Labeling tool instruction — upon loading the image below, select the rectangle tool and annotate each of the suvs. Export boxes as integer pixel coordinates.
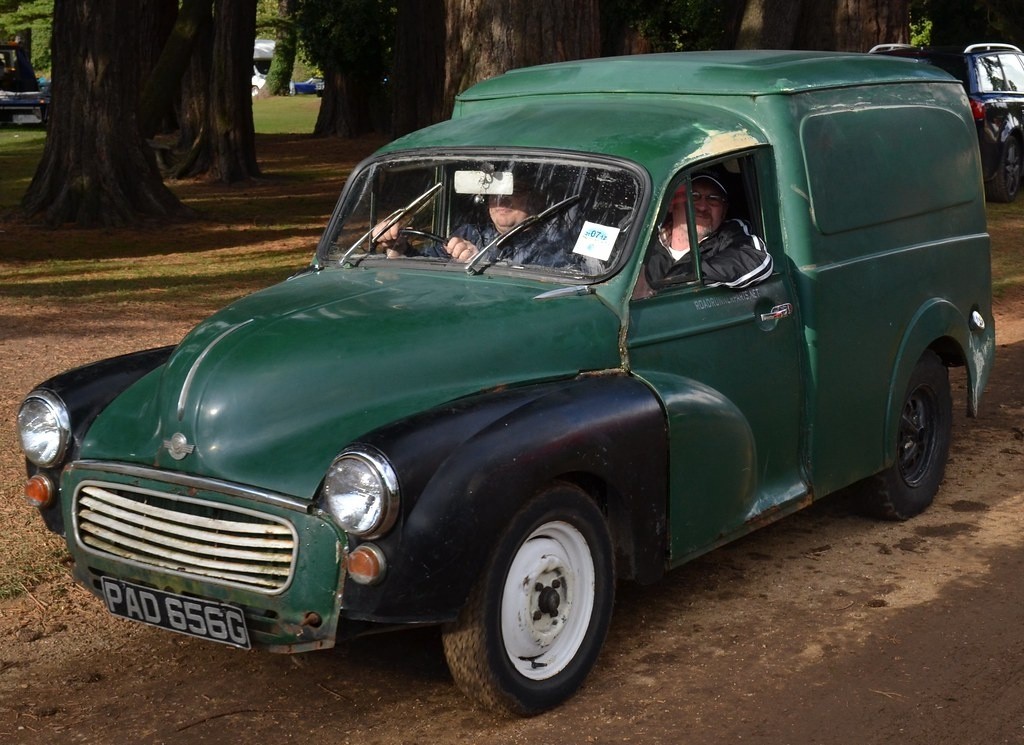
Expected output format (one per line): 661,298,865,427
868,43,1024,203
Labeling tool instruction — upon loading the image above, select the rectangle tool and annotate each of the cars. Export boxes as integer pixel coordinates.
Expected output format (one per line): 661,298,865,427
294,77,324,95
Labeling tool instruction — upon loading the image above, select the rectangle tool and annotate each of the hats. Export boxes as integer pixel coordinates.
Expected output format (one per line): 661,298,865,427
675,167,728,194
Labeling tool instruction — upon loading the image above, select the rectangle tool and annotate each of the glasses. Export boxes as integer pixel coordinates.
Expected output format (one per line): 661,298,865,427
690,190,726,207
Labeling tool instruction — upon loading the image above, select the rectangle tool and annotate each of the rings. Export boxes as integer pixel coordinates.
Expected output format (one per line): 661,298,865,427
467,248,472,253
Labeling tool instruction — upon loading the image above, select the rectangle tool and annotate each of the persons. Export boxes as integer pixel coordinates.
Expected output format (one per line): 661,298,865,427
372,170,589,276
638,168,774,289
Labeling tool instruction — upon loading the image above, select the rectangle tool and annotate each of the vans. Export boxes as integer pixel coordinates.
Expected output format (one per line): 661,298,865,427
17,49,996,719
251,40,295,97
0,42,50,115
